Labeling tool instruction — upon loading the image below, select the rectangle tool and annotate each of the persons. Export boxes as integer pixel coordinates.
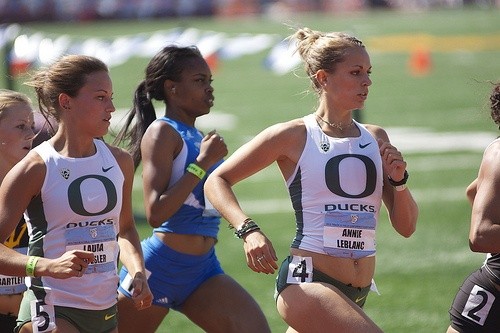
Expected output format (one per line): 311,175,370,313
201,20,420,332
445,77,500,333
1,44,272,333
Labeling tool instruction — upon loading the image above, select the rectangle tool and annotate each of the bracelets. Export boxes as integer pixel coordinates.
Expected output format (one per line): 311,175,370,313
387,170,409,187
391,183,407,191
186,162,206,180
226,218,260,240
25,255,42,278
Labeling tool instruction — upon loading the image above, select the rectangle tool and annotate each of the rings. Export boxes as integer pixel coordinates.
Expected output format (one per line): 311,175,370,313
256,255,265,263
79,265,83,271
78,271,80,277
140,300,144,309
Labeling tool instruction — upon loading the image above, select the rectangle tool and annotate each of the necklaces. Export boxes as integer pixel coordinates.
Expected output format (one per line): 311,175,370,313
314,110,355,130
